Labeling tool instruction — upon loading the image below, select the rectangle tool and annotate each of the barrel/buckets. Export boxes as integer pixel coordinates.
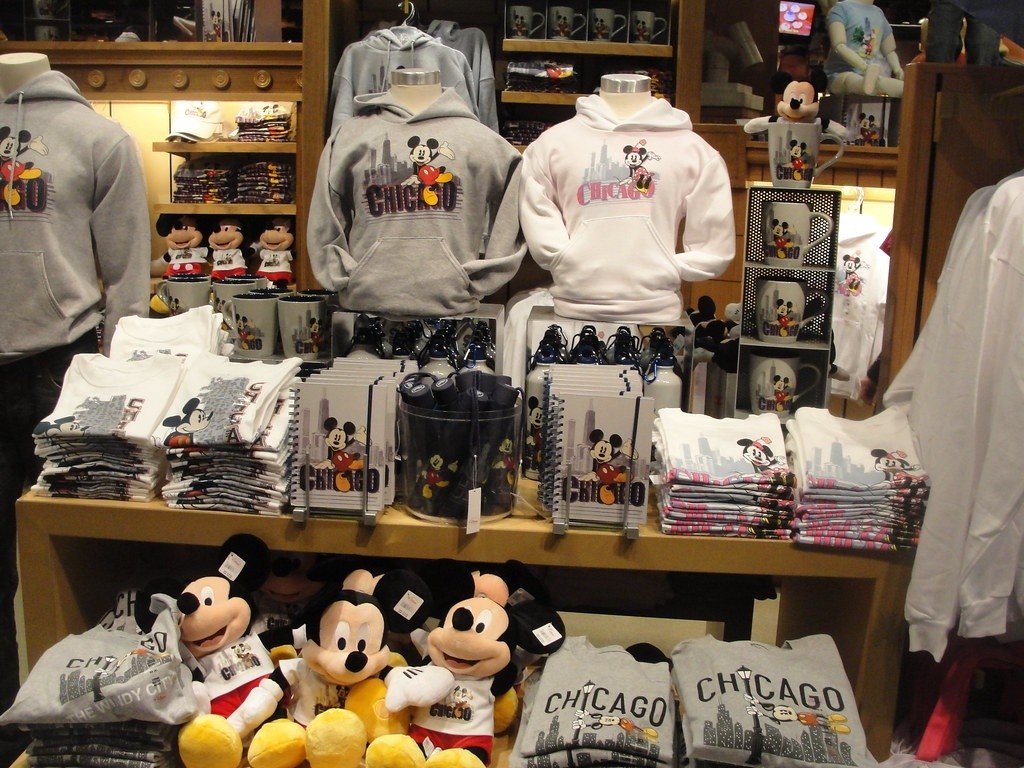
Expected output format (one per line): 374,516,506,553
397,398,520,526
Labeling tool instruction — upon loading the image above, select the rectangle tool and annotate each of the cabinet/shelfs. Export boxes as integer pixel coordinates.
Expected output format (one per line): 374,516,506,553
736,185,839,419
157,48,325,298
501,0,704,155
16,466,914,761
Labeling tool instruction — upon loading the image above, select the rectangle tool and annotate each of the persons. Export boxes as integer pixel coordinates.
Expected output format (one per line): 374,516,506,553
824,0,908,97
517,74,735,323
0,52,152,729
306,68,526,317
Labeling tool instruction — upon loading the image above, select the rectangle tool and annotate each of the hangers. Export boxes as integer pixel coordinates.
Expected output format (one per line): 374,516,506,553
342,4,481,70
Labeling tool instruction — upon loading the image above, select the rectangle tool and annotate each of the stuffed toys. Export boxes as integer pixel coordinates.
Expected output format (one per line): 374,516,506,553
743,69,850,139
150,214,300,291
133,532,565,768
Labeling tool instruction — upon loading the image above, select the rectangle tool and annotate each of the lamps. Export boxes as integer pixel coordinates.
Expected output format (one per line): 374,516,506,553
701,16,769,112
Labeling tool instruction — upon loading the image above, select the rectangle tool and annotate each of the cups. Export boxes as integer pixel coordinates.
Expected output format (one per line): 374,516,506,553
760,200,833,268
548,6,586,42
31,0,69,19
589,7,628,42
507,5,546,39
748,350,823,418
157,270,342,363
33,26,62,42
753,277,829,347
630,10,668,44
768,121,845,193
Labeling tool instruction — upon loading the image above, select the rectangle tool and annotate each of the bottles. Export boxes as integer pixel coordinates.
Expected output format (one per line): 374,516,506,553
347,312,499,393
525,322,682,483
